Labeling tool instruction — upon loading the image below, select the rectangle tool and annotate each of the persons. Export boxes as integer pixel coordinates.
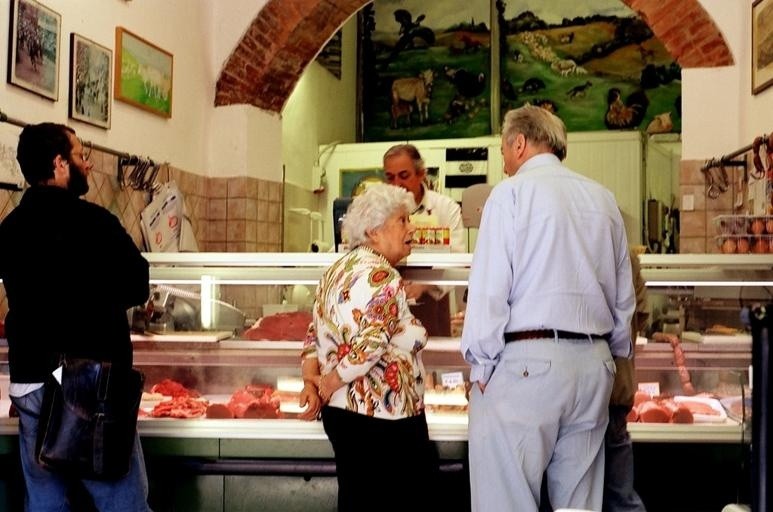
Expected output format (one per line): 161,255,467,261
0,122,151,510
296,179,435,512
382,145,466,253
459,104,638,512
599,239,646,510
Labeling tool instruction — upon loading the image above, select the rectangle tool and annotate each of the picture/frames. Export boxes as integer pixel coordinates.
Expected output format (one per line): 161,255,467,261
8,0,61,104
69,32,114,131
750,0,772,95
114,27,174,120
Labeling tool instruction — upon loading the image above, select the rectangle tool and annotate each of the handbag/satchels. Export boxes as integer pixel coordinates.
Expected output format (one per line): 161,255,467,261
36,353,146,482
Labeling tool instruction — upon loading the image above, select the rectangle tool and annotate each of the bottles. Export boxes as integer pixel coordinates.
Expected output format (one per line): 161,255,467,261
421,228,435,244
411,229,422,244
435,228,449,245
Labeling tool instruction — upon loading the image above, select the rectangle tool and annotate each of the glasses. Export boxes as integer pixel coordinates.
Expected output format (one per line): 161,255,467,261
71,146,93,160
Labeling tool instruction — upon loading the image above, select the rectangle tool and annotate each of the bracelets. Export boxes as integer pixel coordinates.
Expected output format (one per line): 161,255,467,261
316,376,334,402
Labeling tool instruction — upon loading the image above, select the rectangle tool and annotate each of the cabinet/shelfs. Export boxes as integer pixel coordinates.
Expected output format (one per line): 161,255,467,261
0,250,773,512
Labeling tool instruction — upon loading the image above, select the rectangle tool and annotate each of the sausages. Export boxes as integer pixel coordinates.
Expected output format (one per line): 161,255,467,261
651,331,694,396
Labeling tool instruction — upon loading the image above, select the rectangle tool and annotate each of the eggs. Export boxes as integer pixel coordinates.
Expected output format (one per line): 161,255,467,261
750,220,764,235
768,239,772,252
764,219,773,234
722,238,735,253
736,238,748,252
753,239,768,253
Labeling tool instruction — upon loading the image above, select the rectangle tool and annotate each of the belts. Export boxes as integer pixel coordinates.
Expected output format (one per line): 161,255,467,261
504,326,599,342
